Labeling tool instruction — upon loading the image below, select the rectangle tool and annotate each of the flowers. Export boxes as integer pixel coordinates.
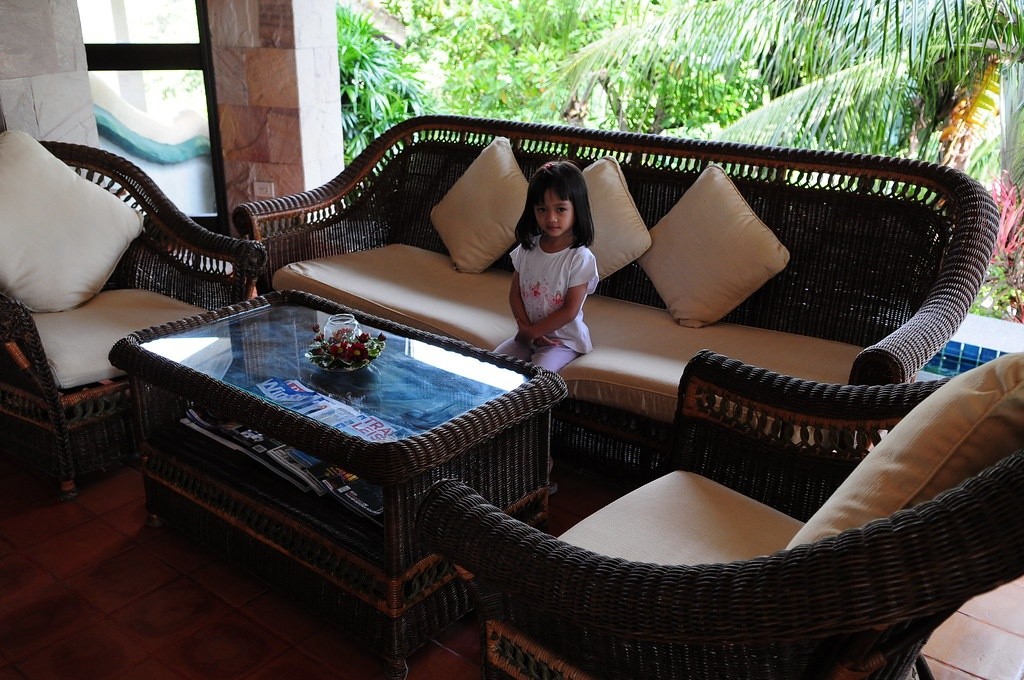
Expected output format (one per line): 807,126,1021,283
303,324,387,372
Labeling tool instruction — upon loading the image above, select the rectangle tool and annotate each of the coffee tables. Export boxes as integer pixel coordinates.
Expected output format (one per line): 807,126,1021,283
107,286,570,680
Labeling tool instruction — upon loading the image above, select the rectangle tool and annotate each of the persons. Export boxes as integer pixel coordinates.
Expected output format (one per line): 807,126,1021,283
492,160,599,386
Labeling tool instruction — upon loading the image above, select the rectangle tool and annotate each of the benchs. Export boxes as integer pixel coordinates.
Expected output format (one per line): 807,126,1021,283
229,116,999,482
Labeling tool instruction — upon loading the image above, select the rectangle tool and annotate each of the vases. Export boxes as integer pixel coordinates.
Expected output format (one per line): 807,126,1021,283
324,314,362,342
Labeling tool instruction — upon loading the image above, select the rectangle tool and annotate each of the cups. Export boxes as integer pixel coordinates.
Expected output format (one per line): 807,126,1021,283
324,314,363,344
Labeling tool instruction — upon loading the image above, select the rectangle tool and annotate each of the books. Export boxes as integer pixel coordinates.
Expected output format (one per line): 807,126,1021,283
180,376,468,530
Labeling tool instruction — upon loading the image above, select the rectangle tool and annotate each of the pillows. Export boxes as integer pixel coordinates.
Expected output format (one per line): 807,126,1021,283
783,352,1024,551
581,155,653,282
430,136,529,275
0,129,144,313
635,164,791,328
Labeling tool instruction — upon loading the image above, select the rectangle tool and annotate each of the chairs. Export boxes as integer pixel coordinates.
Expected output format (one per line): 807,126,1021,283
409,348,1024,680
1,140,269,503
